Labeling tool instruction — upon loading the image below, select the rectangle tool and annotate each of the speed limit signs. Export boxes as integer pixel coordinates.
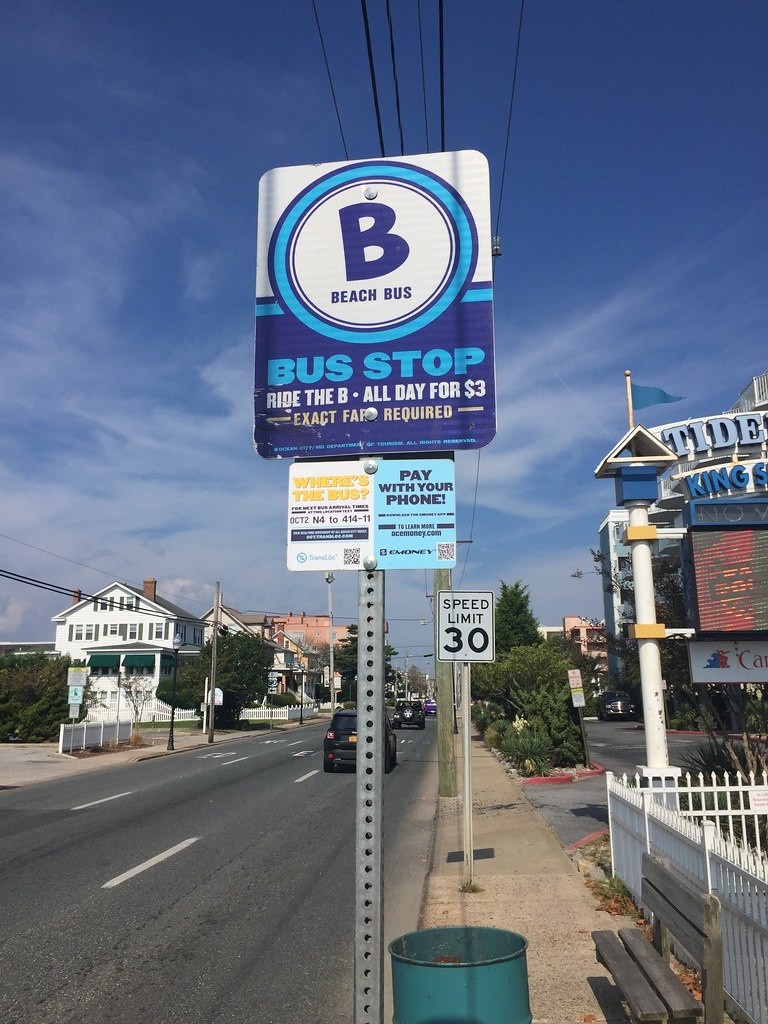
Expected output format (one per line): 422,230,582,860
434,589,496,664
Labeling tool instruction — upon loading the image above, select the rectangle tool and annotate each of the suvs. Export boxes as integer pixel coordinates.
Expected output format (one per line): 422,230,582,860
323,707,399,774
392,700,427,729
597,690,637,721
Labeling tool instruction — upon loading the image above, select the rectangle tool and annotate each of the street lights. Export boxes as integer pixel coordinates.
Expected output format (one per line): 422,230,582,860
165,632,183,750
298,664,306,725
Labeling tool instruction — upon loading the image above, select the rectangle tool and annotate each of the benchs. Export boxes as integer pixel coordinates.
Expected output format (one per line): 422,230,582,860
591,851,723,1023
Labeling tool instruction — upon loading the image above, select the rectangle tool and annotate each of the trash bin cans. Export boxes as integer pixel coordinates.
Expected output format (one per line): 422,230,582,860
388,923,532,1024
